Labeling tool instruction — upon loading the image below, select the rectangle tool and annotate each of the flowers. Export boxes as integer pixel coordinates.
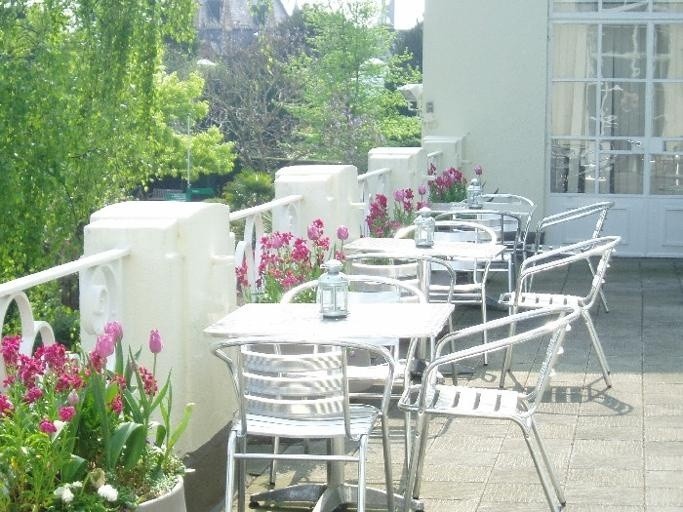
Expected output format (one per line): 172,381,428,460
236,219,352,304
0,323,183,512
427,164,483,202
366,187,431,236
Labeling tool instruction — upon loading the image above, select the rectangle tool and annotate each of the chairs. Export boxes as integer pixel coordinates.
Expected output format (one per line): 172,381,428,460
457,192,530,273
435,210,523,304
213,334,395,508
400,305,581,511
342,251,463,387
278,275,435,452
528,198,615,315
390,220,499,369
498,236,623,389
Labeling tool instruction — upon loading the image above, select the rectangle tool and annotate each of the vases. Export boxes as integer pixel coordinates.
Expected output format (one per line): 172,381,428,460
132,476,185,511
